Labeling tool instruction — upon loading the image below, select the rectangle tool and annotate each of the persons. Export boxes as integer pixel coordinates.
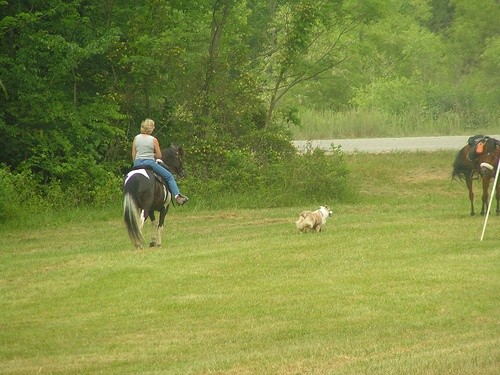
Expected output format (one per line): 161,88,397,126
131,118,189,206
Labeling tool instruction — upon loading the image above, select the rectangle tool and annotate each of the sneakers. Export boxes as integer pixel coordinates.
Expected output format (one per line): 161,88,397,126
175,194,190,206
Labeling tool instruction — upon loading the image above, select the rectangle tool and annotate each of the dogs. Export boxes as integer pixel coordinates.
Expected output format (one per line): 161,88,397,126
296,205,333,234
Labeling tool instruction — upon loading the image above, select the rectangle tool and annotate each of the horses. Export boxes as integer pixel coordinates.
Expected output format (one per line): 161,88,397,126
451,135,500,216
123,143,185,251
